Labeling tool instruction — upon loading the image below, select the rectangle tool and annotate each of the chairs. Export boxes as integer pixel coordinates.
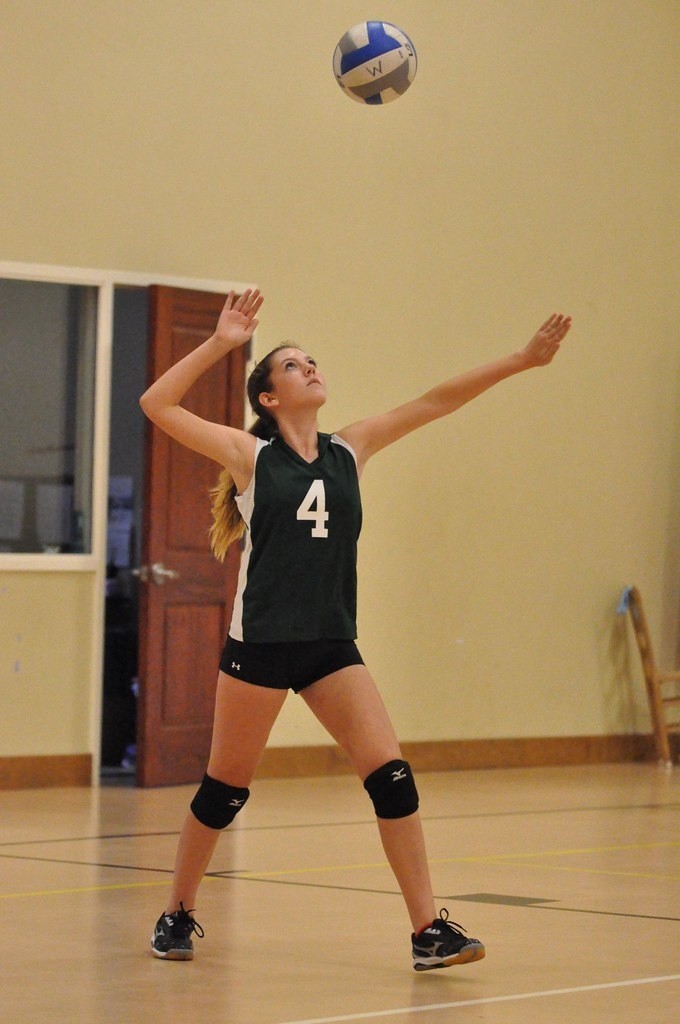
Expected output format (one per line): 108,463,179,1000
627,586,680,769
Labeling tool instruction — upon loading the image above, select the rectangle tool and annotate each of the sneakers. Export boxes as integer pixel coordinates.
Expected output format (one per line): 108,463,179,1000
411,908,485,971
151,901,204,960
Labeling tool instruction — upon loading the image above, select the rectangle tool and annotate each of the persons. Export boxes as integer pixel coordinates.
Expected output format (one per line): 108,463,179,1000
138,287,573,971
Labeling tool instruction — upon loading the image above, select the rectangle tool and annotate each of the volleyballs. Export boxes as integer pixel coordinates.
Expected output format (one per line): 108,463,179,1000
332,20,418,105
